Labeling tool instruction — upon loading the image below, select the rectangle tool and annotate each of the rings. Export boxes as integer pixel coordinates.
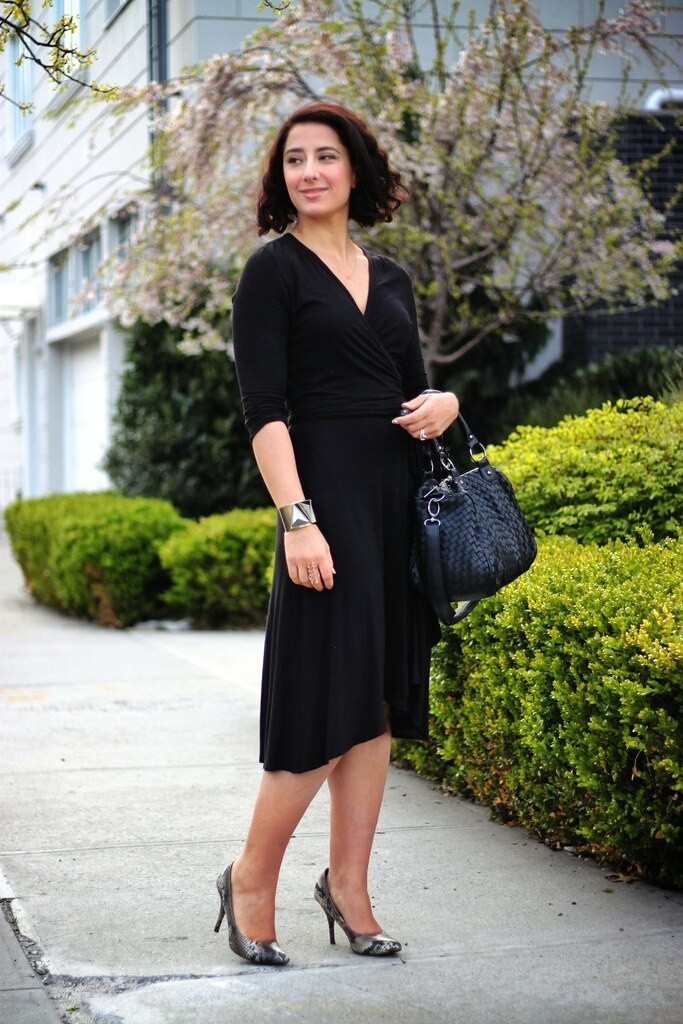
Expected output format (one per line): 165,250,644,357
419,429,426,441
307,560,318,584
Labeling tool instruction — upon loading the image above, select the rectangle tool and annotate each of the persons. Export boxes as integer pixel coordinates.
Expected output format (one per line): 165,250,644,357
216,101,464,970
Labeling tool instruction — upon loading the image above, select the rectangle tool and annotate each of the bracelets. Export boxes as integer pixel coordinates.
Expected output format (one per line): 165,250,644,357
278,499,319,533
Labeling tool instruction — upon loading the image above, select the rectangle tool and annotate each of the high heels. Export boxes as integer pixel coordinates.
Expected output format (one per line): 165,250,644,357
213,861,290,966
314,868,401,957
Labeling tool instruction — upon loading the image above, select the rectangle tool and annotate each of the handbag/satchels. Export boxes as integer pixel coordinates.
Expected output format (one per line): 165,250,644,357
408,387,539,626
